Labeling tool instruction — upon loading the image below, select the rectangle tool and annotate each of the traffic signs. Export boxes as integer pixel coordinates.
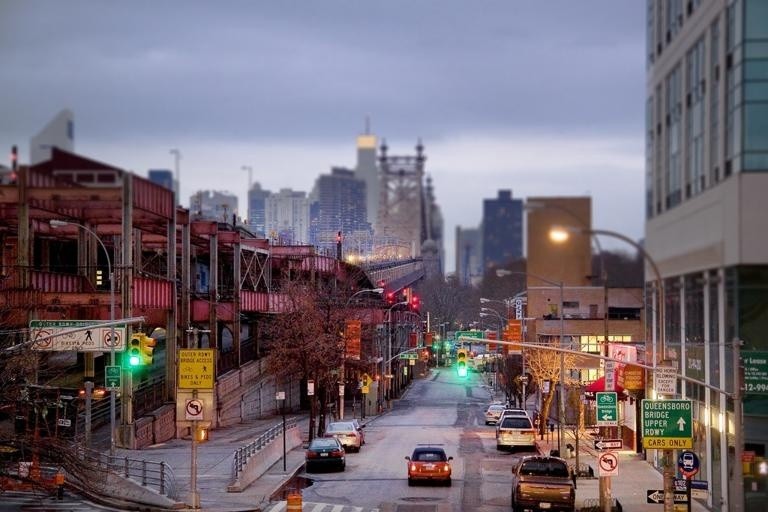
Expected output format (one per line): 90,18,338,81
596,391,618,427
594,439,623,450
105,366,122,392
640,399,694,449
647,479,709,512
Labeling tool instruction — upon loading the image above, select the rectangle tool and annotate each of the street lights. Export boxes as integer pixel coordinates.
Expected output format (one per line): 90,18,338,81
337,288,384,421
547,224,675,512
478,297,527,411
496,268,568,459
50,219,116,463
381,301,408,409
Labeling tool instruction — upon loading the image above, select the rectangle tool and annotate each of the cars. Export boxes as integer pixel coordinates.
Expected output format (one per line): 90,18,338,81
404,446,453,487
323,418,366,454
483,404,537,452
510,454,576,512
303,436,347,475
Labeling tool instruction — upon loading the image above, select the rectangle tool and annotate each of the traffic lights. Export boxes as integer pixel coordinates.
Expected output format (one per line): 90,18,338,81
457,348,468,378
129,334,142,368
